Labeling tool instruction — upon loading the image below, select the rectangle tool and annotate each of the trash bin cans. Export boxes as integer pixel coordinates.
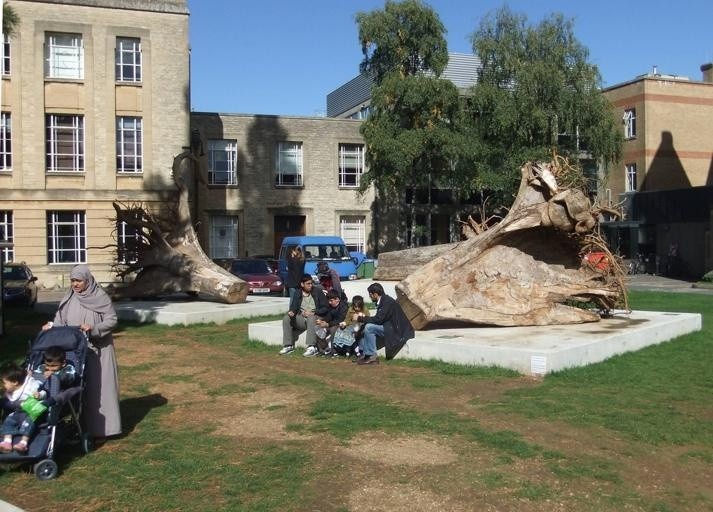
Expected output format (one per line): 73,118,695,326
357,260,374,279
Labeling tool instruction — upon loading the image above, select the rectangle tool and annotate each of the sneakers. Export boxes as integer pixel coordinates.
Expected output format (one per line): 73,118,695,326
277,344,295,355
0,440,27,454
301,346,319,357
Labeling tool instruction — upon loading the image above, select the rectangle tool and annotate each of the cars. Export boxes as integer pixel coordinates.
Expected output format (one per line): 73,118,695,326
1,260,39,309
213,254,285,296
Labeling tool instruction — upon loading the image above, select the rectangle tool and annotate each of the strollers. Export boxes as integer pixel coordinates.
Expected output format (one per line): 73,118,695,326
0,325,95,482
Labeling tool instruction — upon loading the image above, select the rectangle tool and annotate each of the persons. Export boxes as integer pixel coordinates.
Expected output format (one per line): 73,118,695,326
331,295,370,360
27,345,77,389
287,247,306,307
351,282,415,365
315,288,348,357
0,365,50,453
42,264,122,445
277,273,330,358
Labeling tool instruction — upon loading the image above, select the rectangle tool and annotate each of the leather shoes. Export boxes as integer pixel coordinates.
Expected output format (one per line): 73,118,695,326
350,353,378,366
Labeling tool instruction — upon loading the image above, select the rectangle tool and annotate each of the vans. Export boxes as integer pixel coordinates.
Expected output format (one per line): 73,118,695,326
278,234,364,296
